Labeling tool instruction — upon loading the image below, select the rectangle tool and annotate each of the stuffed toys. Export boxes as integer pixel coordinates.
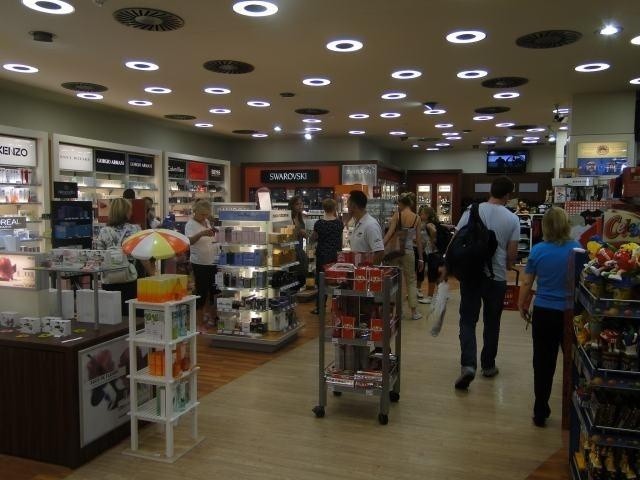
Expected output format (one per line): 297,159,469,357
569,236,640,480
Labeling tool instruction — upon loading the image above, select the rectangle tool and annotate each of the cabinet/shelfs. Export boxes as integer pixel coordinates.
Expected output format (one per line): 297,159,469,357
562,246,640,479
55,136,161,287
367,179,399,238
166,152,228,293
1,248,199,464
248,186,336,210
297,211,324,297
211,205,305,347
0,128,47,254
515,214,544,267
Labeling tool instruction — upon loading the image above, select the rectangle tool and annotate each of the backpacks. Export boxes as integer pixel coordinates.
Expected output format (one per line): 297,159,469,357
101,223,138,285
425,220,453,255
443,202,498,282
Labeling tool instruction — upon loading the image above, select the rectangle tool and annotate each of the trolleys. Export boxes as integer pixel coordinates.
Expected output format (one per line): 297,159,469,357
311,266,402,424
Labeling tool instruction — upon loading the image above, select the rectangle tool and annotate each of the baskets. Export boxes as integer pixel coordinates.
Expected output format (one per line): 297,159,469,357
502,268,535,312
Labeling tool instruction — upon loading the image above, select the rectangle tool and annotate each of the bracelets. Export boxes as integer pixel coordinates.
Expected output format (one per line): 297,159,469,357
419,260,424,262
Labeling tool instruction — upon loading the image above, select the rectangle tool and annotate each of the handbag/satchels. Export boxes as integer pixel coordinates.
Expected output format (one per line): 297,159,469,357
426,279,449,337
384,210,408,262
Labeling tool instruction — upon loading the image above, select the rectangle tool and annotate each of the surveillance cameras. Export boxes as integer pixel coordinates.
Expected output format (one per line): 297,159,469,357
553,113,564,119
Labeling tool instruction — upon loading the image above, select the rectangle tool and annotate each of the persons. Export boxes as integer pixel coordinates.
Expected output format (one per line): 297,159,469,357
505,199,519,214
310,198,344,314
438,175,520,390
346,190,385,265
381,192,425,320
185,200,217,324
286,196,309,292
518,207,585,427
417,207,439,304
418,205,430,298
98,189,161,317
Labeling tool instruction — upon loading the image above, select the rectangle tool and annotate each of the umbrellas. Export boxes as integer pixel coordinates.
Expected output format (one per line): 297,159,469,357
121,228,191,278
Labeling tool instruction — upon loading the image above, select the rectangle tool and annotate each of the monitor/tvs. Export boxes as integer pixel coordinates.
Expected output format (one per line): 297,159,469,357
486,149,527,175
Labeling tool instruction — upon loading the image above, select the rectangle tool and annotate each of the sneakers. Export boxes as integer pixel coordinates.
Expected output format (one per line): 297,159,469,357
412,312,422,320
417,296,433,304
416,288,424,298
455,366,476,390
481,368,498,377
